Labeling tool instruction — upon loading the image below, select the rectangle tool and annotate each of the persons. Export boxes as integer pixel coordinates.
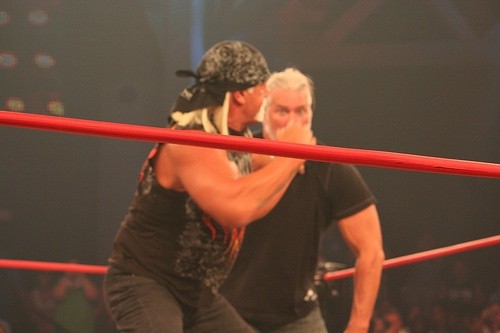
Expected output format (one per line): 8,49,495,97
0,256,113,333
371,259,500,333
103,39,317,333
218,66,385,333
